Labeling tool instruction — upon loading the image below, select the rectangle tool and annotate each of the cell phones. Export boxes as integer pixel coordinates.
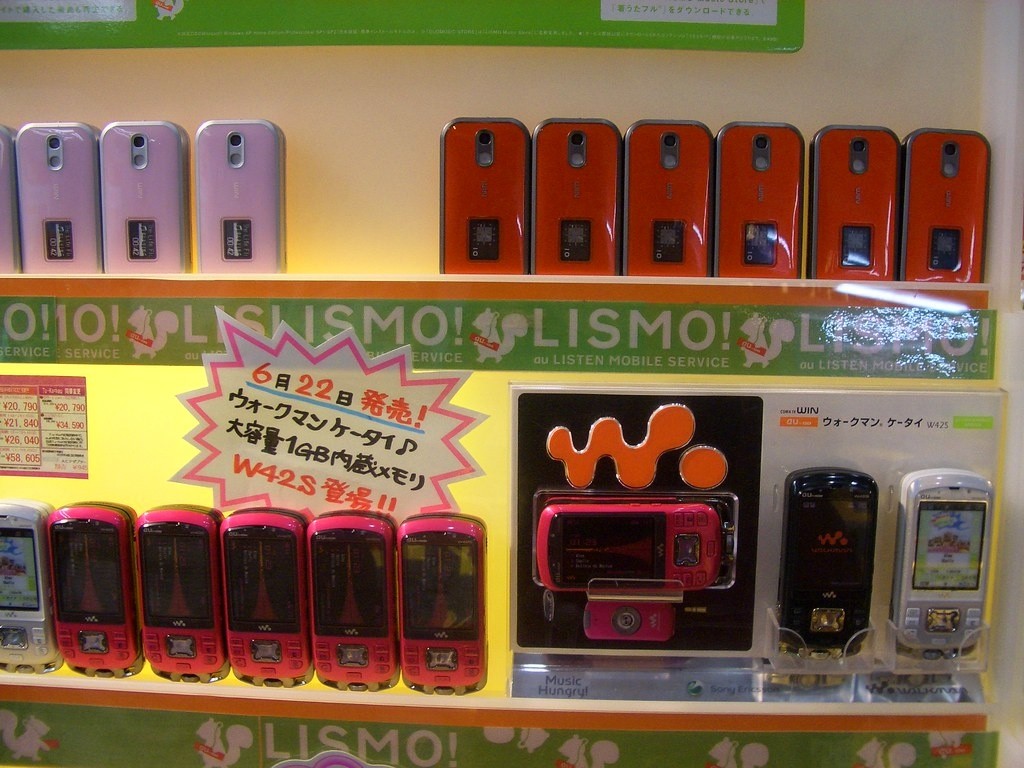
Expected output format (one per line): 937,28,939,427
777,467,880,649
0,499,486,697
1,118,287,277
892,468,997,648
536,496,720,595
435,119,990,285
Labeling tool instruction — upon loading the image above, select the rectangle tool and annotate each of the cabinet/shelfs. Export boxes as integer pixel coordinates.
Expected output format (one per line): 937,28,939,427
0,273,1012,767
